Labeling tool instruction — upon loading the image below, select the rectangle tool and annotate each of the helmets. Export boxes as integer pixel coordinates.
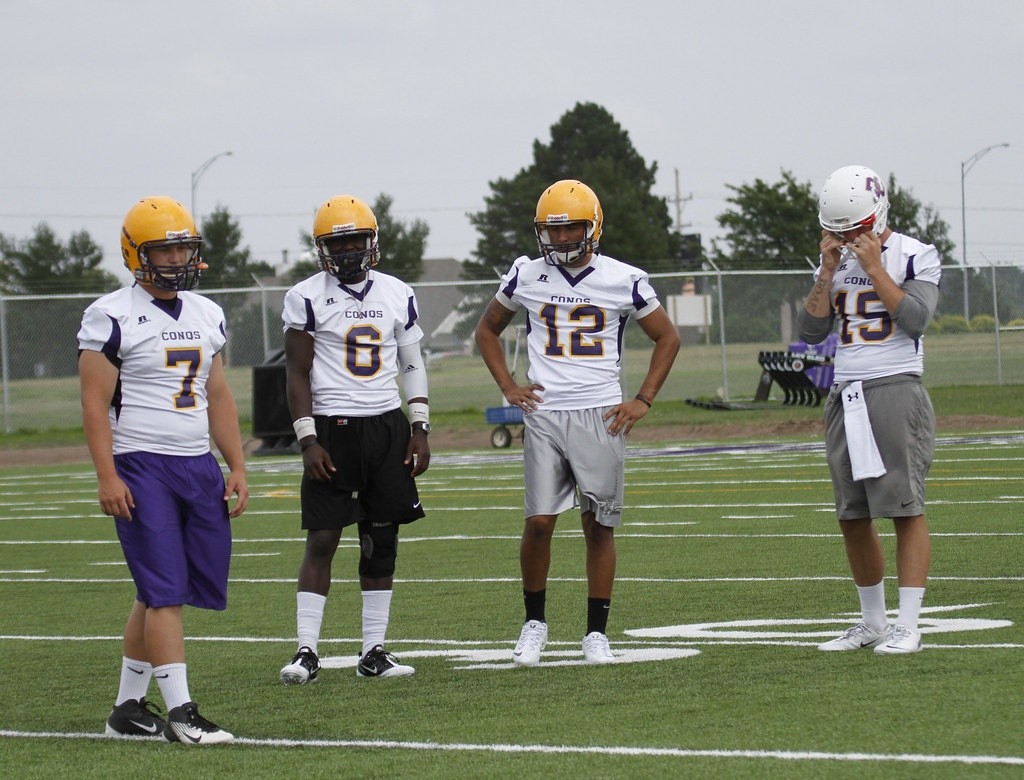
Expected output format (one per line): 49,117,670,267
120,196,208,286
818,165,887,232
312,194,380,272
534,179,604,256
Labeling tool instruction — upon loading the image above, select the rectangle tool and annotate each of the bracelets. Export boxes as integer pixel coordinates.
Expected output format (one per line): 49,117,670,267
300,439,316,453
635,394,652,408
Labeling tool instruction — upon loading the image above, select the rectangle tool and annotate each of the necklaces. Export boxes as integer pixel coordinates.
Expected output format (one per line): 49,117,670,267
345,273,367,314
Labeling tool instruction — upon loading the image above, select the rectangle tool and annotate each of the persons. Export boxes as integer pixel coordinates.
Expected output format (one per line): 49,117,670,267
278,193,433,685
796,164,942,656
76,195,252,747
473,178,682,665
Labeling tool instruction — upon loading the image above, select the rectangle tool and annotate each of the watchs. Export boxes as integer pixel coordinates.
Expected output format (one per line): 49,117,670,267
412,422,432,434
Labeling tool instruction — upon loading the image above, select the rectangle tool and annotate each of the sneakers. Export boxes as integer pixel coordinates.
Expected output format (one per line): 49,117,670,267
104,696,170,742
874,625,923,655
279,646,321,685
818,623,893,651
356,644,416,678
582,632,616,663
162,702,234,744
512,620,548,665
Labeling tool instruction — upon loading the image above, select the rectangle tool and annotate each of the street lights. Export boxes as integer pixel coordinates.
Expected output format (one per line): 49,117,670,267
190,150,232,218
961,143,1011,265
